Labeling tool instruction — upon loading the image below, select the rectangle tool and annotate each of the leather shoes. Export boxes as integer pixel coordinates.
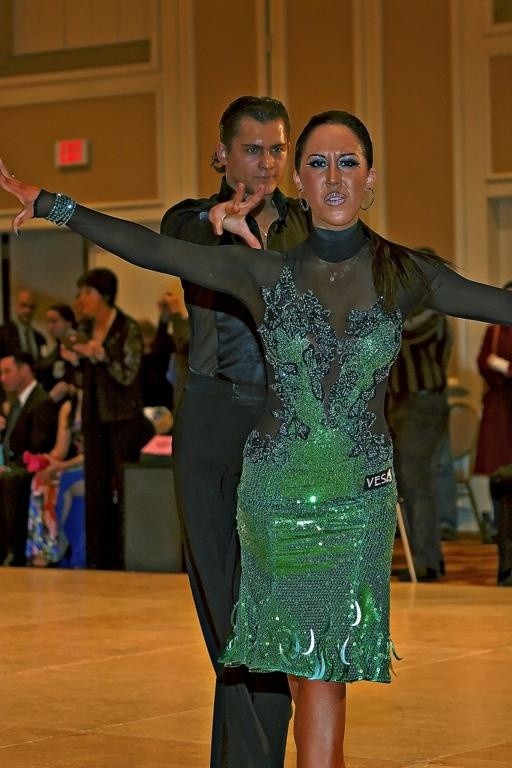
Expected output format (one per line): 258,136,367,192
397,561,445,583
498,568,512,585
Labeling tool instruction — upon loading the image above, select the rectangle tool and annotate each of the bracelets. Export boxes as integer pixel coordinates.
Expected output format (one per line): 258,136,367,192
46,191,78,230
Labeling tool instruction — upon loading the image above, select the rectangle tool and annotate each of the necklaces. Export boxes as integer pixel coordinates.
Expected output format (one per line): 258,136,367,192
322,251,363,283
258,220,269,238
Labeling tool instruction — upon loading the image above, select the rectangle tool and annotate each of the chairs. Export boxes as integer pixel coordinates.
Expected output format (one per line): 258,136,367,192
397,376,480,582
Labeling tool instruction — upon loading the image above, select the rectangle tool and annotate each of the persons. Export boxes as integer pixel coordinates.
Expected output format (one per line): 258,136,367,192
0,106,511,768
1,264,190,570
155,98,315,768
472,278,511,587
383,245,457,583
